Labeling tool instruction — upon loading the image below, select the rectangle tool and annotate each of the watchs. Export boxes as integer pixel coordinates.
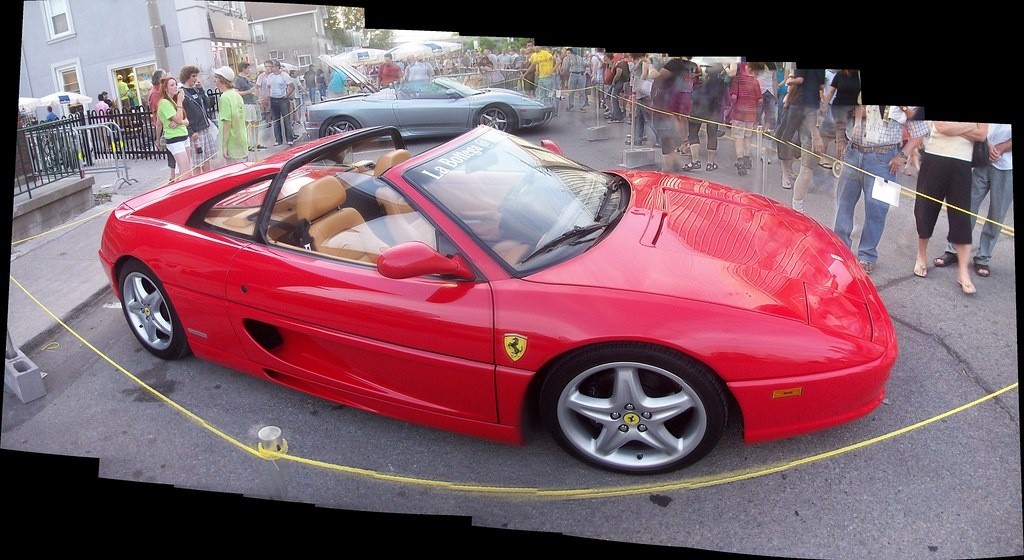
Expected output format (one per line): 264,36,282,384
902,106,908,111
900,150,908,158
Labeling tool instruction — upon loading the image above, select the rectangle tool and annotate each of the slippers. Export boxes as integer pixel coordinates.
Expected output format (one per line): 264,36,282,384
957,280,976,293
914,264,927,277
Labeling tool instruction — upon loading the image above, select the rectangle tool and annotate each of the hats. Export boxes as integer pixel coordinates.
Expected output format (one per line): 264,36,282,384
102,92,108,95
127,73,134,77
127,82,134,86
117,75,123,79
212,66,234,82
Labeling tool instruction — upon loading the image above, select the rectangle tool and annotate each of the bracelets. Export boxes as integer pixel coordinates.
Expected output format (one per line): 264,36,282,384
836,137,846,143
177,105,182,109
854,125,861,127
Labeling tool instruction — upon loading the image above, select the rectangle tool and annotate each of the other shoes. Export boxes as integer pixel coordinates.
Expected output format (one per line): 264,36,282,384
567,102,662,149
287,141,293,145
273,142,282,146
860,261,873,274
674,130,832,189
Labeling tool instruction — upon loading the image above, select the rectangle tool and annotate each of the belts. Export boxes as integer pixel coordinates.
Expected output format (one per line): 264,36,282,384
848,141,895,154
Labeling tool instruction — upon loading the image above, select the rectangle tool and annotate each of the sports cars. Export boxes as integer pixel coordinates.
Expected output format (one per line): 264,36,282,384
97,125,900,476
303,54,555,151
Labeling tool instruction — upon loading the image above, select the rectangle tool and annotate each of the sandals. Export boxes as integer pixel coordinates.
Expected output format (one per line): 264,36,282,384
248,146,260,152
974,262,990,277
258,144,267,149
934,253,958,266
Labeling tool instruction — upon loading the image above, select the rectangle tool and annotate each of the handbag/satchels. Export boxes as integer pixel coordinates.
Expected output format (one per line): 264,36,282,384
159,135,167,148
208,118,219,140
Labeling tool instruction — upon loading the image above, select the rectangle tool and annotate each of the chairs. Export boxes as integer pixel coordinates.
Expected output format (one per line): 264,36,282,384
296,176,390,264
389,81,411,99
373,149,437,250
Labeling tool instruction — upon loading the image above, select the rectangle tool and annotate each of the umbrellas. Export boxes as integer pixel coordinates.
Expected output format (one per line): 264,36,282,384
387,40,462,61
334,47,388,65
18,89,91,115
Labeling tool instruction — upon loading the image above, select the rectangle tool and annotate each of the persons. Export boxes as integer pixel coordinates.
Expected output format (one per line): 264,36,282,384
289,41,924,217
256,59,274,128
155,77,193,181
116,73,140,107
213,65,250,166
179,65,218,176
833,103,930,273
914,121,989,294
376,54,404,91
97,91,118,153
235,61,267,151
934,122,1012,278
268,61,295,146
147,68,167,179
47,106,59,120
